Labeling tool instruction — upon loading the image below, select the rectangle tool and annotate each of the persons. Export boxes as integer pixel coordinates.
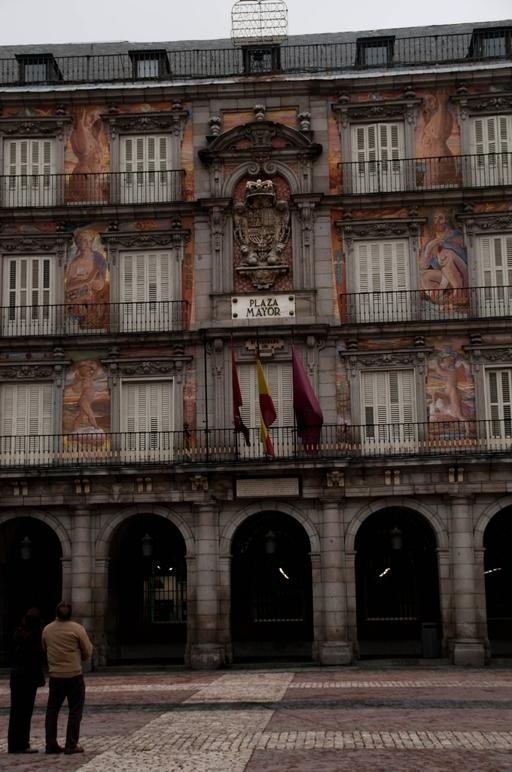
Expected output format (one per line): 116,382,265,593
7,606,48,754
419,207,467,304
41,600,94,753
71,359,101,430
65,226,107,319
419,91,457,186
68,103,106,201
432,352,471,438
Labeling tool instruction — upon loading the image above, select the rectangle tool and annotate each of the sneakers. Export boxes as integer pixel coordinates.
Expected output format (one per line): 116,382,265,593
65,745,84,753
46,746,65,753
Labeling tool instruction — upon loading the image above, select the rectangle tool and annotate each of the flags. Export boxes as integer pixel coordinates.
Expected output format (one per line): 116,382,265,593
290,343,323,455
255,346,277,461
232,338,252,447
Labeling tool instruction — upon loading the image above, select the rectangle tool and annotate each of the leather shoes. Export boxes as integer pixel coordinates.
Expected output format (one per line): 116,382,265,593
8,747,39,754
